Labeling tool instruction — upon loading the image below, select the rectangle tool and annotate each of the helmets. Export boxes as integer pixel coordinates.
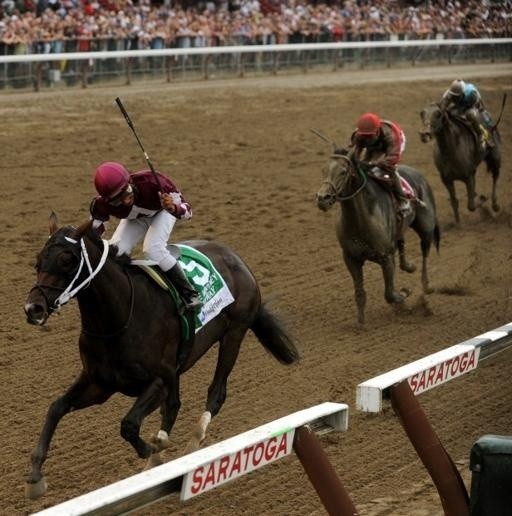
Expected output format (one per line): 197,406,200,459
356,114,381,134
447,79,465,97
94,161,130,201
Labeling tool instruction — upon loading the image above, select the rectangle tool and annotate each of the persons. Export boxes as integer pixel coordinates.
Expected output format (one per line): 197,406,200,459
438,79,490,143
0,0,512,89
347,113,412,217
89,161,206,312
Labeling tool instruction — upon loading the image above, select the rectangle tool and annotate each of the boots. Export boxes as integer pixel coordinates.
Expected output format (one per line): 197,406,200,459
166,260,204,311
389,167,411,215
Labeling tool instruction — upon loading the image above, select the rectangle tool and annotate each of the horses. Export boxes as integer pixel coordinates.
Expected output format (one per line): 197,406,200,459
21,211,299,502
419,90,509,222
316,143,440,326
